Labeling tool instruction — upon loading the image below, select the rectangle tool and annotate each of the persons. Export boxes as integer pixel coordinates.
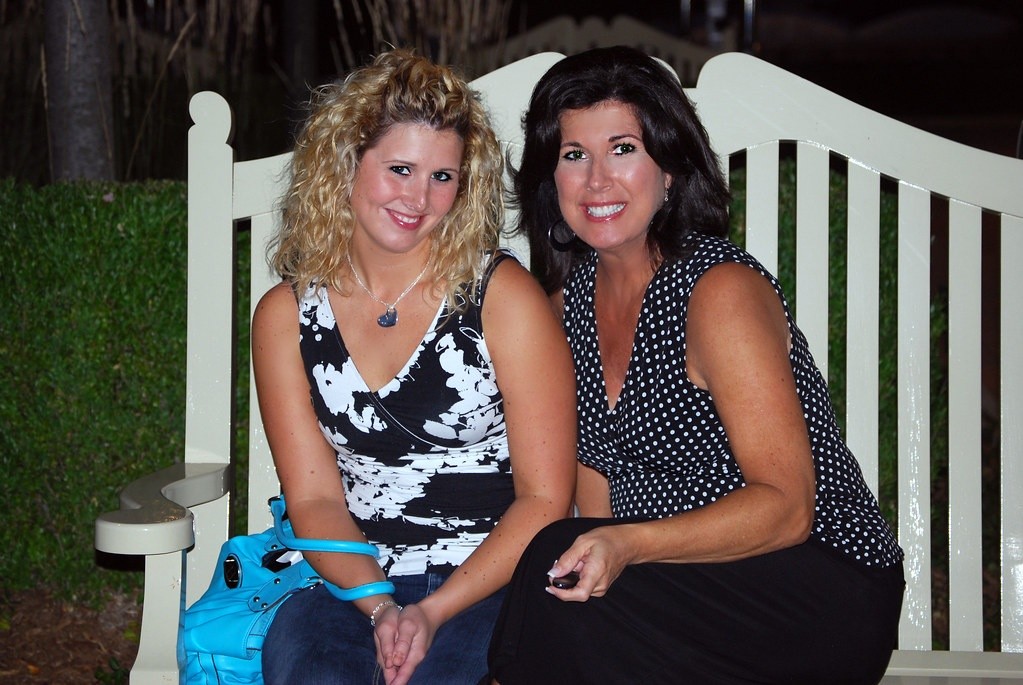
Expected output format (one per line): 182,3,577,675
487,45,906,685
251,51,577,685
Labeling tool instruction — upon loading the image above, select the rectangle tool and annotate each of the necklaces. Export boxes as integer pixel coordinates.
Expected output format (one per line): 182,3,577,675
347,248,435,328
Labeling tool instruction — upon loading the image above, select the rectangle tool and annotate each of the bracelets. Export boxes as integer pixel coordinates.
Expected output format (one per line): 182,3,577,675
370,601,403,624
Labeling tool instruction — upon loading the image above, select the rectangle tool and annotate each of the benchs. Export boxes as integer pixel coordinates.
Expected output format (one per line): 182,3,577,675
95,53,1022,685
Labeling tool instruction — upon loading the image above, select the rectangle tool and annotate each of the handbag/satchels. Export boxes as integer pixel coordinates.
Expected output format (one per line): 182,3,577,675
177,495,394,685
